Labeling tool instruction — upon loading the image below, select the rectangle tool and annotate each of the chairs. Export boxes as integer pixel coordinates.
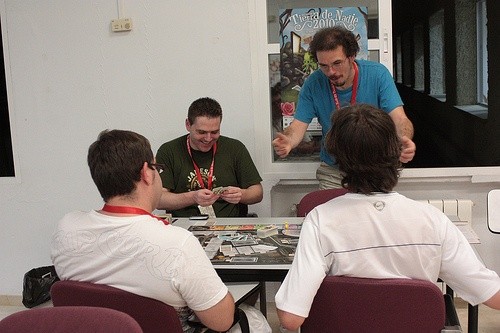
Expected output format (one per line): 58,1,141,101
299,276,463,333
0,280,251,333
296,188,350,218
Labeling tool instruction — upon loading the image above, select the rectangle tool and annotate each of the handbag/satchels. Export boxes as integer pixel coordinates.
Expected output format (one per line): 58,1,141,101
23,265,60,308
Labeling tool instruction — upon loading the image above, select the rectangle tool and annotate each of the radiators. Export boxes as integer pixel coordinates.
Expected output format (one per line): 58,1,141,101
290,199,476,298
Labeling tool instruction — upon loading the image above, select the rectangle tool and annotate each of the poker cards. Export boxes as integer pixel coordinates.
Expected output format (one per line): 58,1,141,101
211,186,230,193
220,232,279,257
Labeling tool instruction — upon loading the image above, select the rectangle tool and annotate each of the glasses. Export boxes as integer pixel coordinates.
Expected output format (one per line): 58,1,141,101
139,163,166,174
317,56,348,71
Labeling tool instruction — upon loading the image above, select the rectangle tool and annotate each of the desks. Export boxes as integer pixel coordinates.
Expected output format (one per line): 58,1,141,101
156,215,485,333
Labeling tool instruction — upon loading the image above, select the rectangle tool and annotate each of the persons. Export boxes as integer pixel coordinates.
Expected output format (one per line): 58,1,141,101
273,26,415,190
50,129,271,333
152,97,264,217
274,104,500,330
